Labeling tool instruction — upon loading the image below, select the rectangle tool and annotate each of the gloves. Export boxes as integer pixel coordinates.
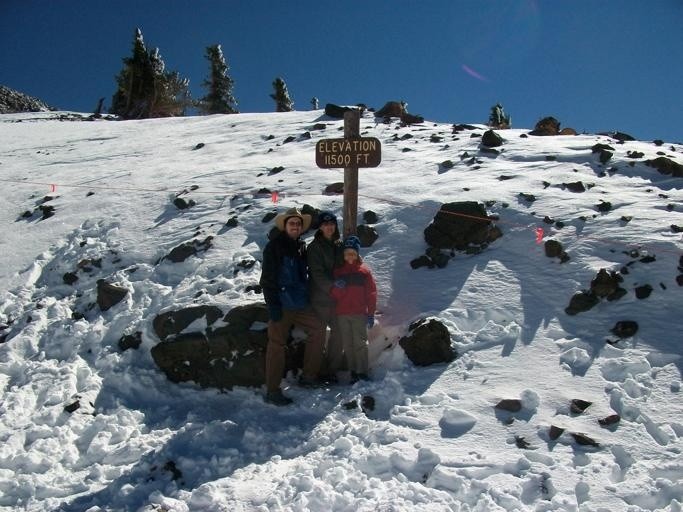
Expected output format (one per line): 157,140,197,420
367,316,375,328
335,279,345,290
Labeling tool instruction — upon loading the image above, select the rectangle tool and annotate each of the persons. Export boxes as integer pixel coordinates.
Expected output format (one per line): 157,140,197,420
330,236,376,385
307,213,344,384
260,208,329,404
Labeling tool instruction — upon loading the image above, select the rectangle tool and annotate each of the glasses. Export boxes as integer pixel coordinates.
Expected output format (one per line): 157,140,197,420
287,220,302,227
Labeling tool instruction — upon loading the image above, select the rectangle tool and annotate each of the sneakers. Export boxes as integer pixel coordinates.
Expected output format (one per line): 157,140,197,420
264,389,293,406
297,373,370,388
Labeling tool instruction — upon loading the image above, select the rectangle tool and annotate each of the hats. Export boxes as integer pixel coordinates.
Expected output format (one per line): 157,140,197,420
276,207,313,234
319,212,337,226
342,236,361,254
